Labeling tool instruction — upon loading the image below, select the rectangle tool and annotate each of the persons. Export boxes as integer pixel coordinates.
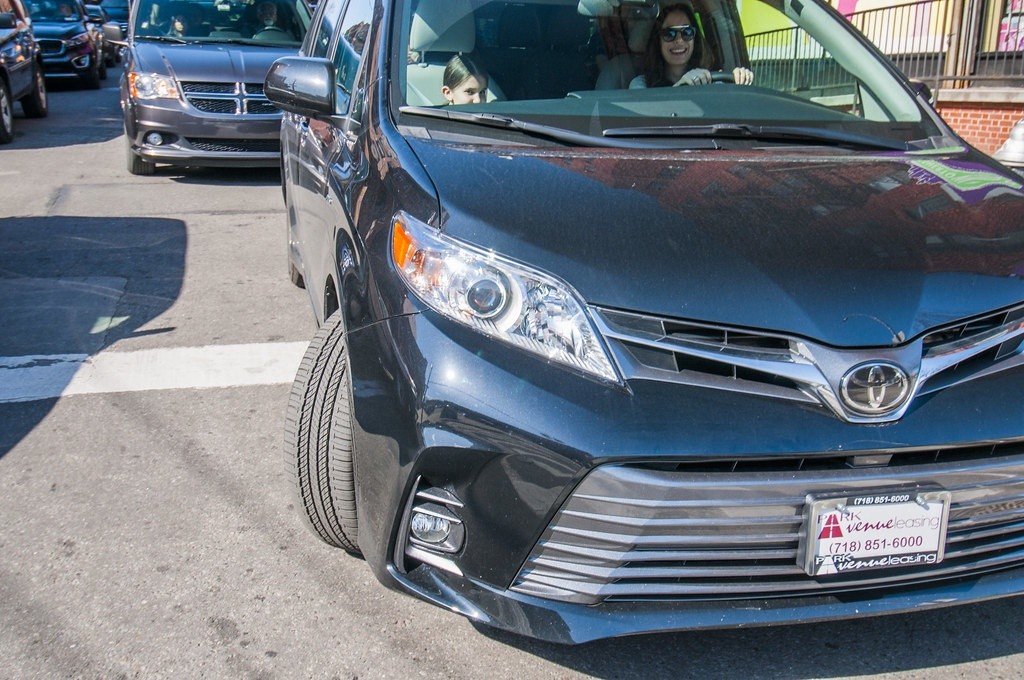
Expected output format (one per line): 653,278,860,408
441,53,491,107
252,1,295,39
588,4,755,91
167,14,192,37
59,3,78,17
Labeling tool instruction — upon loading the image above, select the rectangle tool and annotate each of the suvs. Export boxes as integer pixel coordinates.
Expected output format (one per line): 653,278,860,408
263,0,1024,647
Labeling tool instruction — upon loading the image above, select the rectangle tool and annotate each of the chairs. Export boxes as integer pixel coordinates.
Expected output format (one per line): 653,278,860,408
595,20,655,91
406,0,505,106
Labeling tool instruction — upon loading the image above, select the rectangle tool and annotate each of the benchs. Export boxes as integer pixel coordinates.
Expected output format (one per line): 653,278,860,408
484,6,594,100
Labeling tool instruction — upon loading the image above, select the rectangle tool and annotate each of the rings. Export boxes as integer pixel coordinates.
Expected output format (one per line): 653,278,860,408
693,75,697,80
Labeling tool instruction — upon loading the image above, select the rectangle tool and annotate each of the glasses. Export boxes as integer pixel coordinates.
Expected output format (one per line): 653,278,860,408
659,26,697,42
356,36,366,43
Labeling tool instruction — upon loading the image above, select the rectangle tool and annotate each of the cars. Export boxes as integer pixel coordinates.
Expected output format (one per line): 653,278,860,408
1,0,316,176
